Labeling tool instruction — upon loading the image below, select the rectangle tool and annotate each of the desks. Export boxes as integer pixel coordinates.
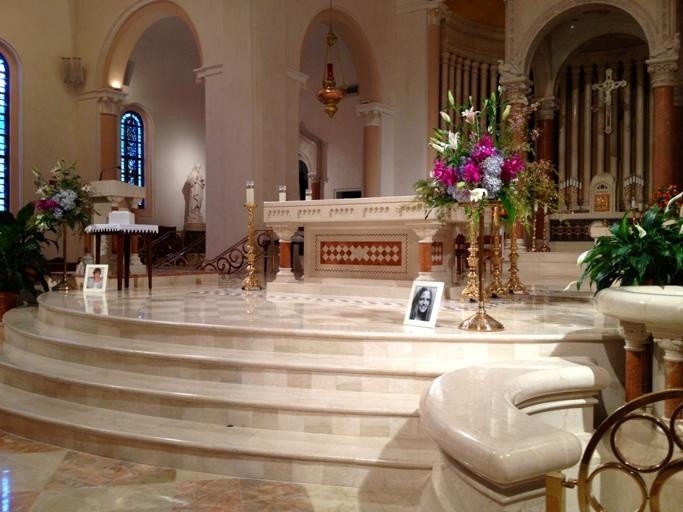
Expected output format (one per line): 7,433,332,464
84,224,159,291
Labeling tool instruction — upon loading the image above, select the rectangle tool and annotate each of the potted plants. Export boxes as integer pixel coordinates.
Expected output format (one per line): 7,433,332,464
31,160,101,234
0,203,53,321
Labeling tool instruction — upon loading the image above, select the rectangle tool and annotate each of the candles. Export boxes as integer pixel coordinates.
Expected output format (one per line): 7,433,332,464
328,62,334,81
279,184,287,201
306,190,313,200
246,179,255,205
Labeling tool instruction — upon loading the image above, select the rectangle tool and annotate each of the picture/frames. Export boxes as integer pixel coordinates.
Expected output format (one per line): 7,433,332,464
403,280,445,329
84,263,109,293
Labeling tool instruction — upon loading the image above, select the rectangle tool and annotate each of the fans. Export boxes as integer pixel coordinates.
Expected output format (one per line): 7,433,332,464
563,185,683,298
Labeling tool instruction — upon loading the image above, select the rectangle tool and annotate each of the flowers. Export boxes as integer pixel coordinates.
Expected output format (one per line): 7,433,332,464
412,84,572,236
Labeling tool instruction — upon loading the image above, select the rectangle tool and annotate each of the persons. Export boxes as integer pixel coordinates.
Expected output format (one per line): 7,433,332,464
86,268,103,289
410,287,432,321
186,163,205,217
